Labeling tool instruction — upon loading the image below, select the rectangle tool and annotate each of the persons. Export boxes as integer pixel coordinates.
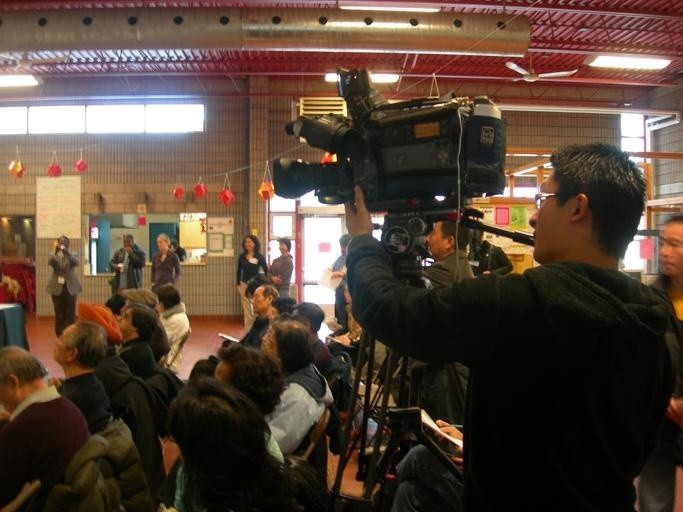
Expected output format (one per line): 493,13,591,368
237,235,294,331
350,316,468,510
46,236,82,338
109,235,145,293
150,233,186,288
1,284,345,511
420,220,512,292
331,235,353,329
640,212,682,511
344,141,677,512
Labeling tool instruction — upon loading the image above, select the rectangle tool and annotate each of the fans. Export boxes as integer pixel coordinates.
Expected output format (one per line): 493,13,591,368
505,55,578,82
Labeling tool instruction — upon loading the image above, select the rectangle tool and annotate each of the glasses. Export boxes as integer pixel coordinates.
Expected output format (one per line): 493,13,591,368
535,193,557,210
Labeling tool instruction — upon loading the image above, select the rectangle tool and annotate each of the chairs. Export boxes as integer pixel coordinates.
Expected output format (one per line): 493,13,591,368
290,407,331,461
163,328,192,369
0,480,41,511
155,352,169,367
326,372,341,387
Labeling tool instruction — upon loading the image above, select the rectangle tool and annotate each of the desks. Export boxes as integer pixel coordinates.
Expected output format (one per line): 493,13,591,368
0,303,30,353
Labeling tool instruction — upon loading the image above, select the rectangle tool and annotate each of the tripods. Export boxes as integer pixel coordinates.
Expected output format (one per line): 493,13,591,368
331,203,535,512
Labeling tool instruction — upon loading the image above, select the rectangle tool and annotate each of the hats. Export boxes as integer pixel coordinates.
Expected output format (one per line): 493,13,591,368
78,295,123,345
